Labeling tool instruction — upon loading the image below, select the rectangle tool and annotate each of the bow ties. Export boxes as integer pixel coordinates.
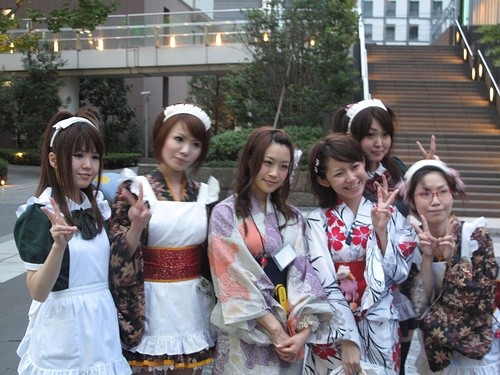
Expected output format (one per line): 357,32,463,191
71,207,98,240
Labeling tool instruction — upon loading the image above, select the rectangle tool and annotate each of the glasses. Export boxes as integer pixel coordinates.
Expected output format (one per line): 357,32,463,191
413,188,453,199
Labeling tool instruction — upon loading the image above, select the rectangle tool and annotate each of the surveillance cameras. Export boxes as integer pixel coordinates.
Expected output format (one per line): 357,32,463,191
140,91,151,95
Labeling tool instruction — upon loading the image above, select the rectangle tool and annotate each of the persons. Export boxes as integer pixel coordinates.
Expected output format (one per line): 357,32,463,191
332,99,439,218
303,133,419,375
108,102,220,375
399,159,500,375
15,111,132,375
208,126,334,375
